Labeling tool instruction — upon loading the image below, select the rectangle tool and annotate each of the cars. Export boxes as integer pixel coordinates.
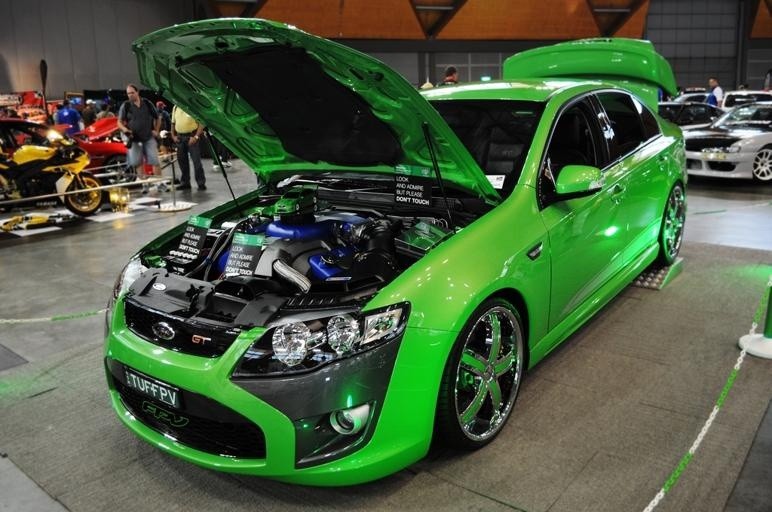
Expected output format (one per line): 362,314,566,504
104,16,688,490
1,117,136,188
657,87,772,132
682,102,772,185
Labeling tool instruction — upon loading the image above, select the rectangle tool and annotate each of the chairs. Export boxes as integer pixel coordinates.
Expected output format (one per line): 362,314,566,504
488,118,533,177
448,109,492,182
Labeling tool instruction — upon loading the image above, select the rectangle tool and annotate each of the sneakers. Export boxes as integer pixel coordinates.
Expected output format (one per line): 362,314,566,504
142,184,149,193
214,162,232,168
158,183,170,191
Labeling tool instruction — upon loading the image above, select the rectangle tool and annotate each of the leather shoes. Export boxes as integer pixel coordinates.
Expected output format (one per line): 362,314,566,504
199,184,207,190
176,185,192,189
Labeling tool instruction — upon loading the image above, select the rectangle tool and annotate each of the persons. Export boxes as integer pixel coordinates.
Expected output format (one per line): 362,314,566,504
434,64,459,89
1,83,232,191
701,76,725,116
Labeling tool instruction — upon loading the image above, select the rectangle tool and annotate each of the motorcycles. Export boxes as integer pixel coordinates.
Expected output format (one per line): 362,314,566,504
0,131,102,216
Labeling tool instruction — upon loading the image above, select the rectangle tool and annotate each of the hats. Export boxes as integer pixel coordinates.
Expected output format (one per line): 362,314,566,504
157,101,166,107
56,99,96,109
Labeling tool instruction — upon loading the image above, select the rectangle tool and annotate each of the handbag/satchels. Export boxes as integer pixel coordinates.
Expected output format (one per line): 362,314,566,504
123,135,133,149
